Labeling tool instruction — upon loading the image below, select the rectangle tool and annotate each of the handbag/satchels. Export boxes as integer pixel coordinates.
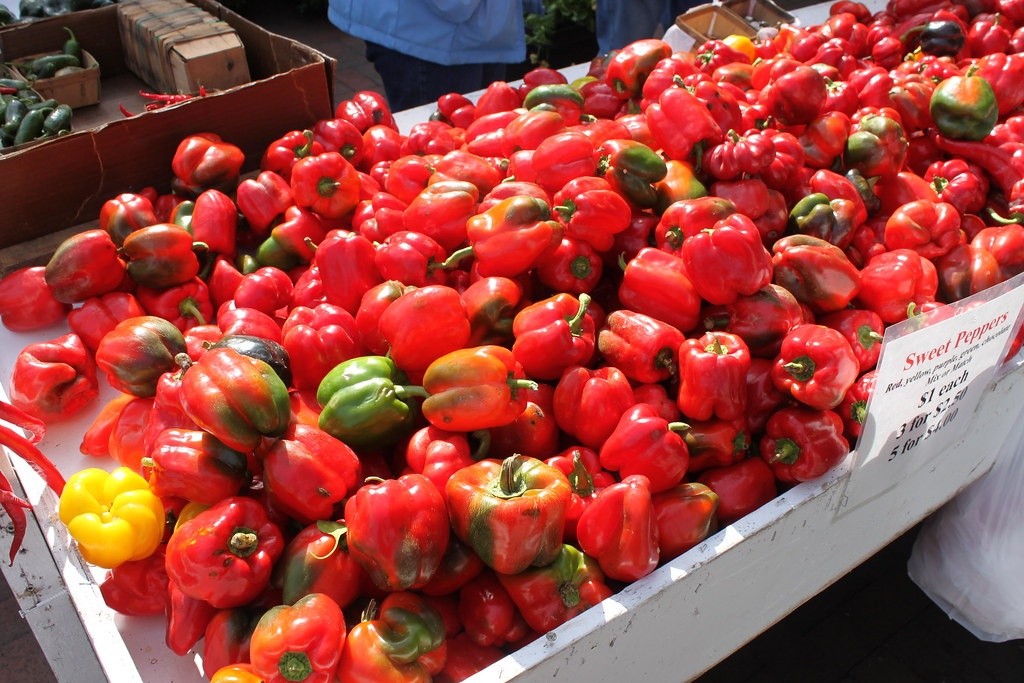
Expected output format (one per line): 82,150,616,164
908,407,1024,644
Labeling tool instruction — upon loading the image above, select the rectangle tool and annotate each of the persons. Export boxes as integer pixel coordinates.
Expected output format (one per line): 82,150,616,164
328,0,544,114
596,0,712,57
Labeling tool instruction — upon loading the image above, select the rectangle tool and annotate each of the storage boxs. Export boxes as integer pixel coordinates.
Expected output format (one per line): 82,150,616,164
116,0,252,95
0,0,338,249
11,49,101,109
0,64,45,103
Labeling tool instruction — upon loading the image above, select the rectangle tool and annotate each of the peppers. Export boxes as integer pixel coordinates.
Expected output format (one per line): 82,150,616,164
0,0,1024,683
